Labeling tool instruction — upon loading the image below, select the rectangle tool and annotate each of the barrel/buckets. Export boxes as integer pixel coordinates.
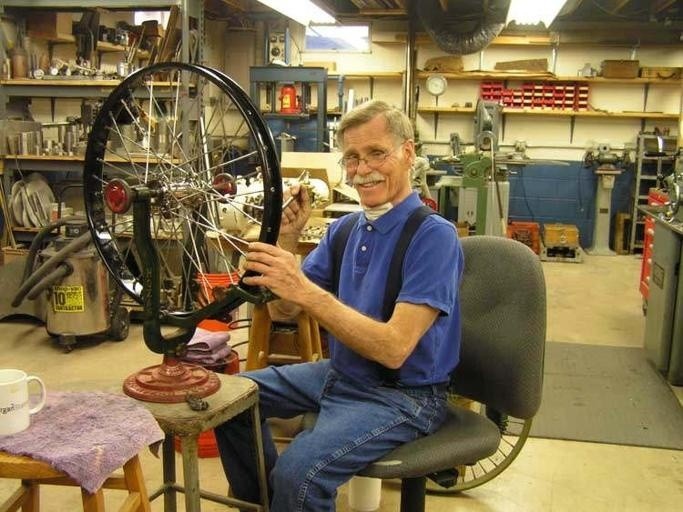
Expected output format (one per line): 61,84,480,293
162,349,241,459
193,270,240,331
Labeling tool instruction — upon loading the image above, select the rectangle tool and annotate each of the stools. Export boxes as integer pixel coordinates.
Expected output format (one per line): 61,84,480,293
0,370,270,512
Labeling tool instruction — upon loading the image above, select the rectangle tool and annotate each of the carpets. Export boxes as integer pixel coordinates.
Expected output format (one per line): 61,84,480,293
494,341,682,448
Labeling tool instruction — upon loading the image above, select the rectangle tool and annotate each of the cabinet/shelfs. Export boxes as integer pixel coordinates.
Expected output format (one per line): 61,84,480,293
1,1,206,322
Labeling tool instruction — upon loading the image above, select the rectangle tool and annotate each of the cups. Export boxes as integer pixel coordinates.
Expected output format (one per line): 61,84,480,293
0,369,46,437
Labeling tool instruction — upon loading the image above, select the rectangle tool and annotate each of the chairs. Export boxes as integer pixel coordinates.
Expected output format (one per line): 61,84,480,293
301,235,546,512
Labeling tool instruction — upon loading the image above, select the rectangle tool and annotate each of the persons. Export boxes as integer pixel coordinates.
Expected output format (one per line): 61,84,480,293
211,97,463,512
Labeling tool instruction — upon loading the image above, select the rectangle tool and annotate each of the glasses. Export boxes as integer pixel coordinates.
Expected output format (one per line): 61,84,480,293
337,149,389,173
